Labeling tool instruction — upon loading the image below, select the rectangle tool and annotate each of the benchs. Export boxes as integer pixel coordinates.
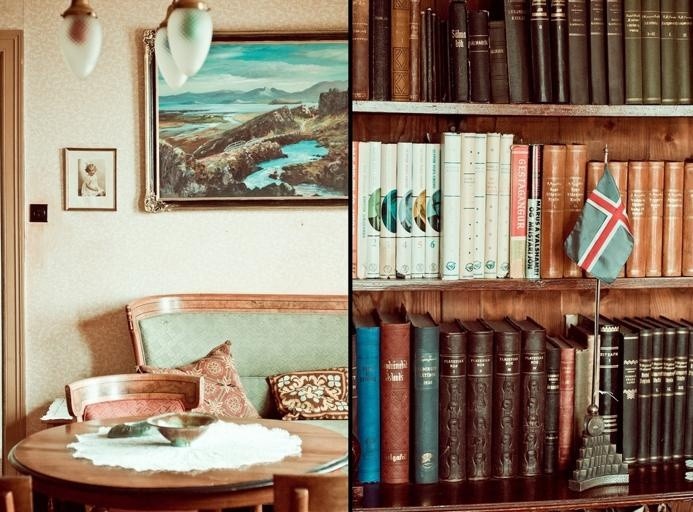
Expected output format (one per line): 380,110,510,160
123,293,350,436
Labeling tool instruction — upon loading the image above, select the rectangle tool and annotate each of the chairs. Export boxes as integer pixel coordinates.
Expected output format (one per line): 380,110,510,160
66,373,204,421
274,472,348,512
1,474,36,512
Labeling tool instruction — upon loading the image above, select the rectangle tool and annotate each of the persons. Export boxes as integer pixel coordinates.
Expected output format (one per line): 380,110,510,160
81,163,105,197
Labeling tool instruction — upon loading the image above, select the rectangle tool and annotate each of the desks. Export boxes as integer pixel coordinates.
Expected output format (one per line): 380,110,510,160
40,397,75,428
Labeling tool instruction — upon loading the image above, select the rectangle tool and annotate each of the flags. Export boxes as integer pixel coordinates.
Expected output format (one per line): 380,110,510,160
561,164,635,284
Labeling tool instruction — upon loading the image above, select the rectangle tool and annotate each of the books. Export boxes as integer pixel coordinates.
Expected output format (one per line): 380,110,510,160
353,132,692,279
352,1,692,106
352,304,692,483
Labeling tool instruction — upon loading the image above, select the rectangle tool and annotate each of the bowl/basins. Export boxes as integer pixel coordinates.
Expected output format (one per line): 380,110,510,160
146,412,219,447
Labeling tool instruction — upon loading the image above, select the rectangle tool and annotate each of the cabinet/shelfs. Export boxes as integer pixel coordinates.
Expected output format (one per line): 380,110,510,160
349,1,692,512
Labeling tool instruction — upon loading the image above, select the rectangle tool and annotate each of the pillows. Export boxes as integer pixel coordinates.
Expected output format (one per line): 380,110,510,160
137,339,261,419
264,366,350,421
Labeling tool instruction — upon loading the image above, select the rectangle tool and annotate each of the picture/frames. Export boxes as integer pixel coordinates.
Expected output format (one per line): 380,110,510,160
139,29,348,212
61,147,117,212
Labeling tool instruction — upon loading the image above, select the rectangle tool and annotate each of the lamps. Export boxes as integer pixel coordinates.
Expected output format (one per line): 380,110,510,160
62,1,212,92
